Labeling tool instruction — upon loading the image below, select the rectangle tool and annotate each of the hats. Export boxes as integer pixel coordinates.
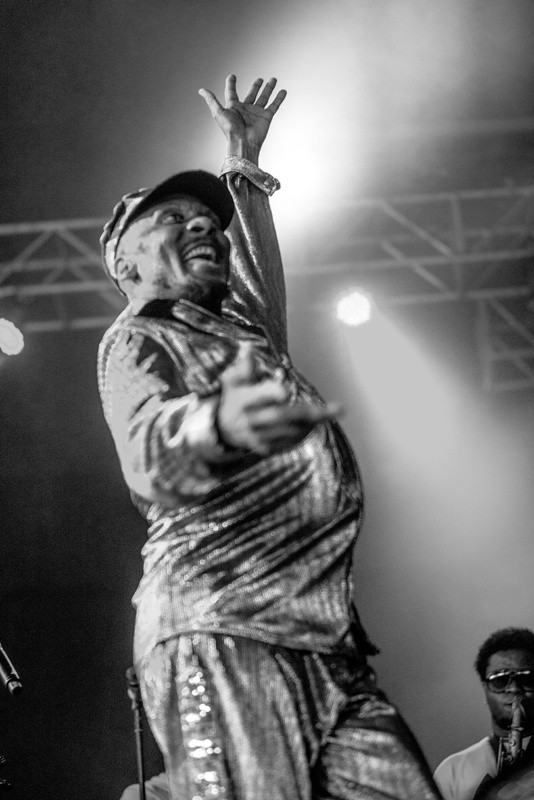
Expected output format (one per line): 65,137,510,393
99,170,234,299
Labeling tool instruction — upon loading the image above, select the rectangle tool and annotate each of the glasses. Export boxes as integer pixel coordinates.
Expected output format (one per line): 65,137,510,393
483,671,534,692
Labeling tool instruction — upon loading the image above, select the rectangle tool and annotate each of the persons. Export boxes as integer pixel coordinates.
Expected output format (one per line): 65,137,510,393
432,627,534,799
95,71,444,800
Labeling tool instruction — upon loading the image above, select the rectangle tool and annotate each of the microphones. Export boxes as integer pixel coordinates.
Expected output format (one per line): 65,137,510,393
125,667,139,688
